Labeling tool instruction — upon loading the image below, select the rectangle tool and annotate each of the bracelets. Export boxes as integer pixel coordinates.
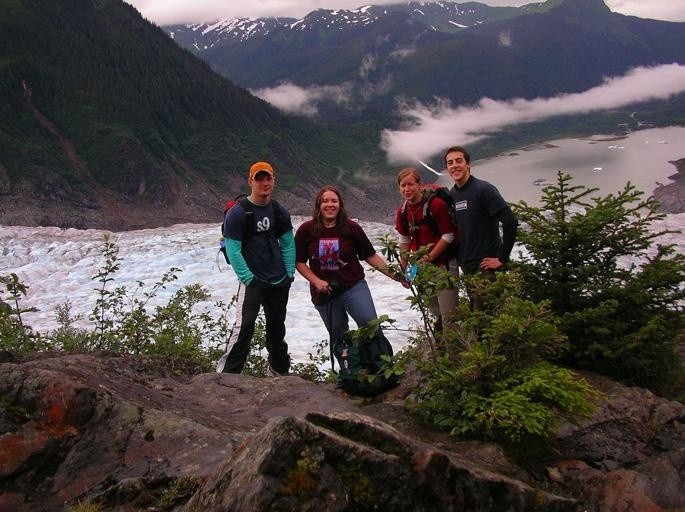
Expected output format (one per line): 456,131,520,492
425,251,432,263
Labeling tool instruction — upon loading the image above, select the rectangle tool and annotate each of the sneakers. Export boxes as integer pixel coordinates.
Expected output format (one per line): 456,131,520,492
267,364,288,377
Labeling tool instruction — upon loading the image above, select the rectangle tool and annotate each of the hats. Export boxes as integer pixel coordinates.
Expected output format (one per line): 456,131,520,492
250,162,274,181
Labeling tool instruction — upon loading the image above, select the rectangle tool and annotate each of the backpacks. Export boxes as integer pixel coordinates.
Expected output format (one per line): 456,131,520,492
401,183,457,236
333,330,401,398
220,194,282,264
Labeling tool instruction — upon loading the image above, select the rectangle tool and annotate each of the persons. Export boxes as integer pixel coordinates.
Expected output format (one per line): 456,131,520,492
294,184,414,372
443,145,518,321
216,159,297,376
396,165,461,360
